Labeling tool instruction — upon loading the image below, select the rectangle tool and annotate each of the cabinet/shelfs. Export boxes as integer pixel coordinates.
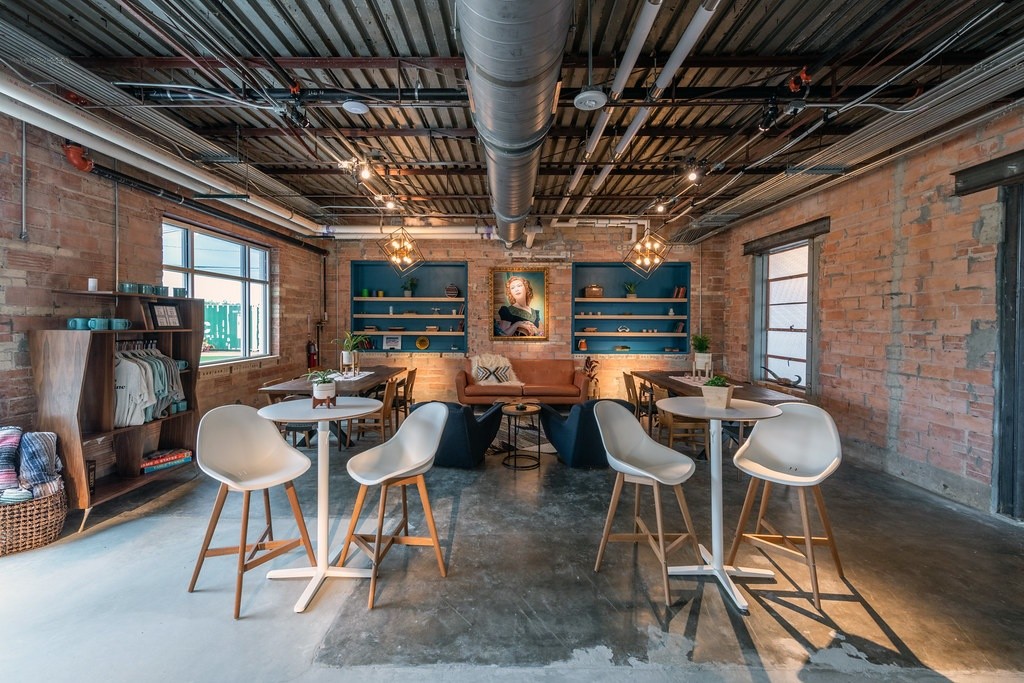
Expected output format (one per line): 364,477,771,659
575,298,687,337
352,297,465,336
28,289,205,547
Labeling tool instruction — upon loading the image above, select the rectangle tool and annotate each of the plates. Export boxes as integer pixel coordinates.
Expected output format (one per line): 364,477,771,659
416,336,429,350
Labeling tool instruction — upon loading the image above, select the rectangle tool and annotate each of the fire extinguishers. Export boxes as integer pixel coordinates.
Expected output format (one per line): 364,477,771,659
308,339,316,368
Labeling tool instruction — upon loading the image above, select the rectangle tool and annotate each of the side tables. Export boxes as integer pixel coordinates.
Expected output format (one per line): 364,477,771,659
502,403,542,470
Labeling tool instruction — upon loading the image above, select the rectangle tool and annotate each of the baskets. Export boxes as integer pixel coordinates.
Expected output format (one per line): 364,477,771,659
0,488,67,556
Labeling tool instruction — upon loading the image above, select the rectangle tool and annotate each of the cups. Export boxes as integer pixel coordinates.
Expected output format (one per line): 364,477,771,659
362,288,369,297
378,291,384,297
88,278,97,291
172,401,187,414
119,283,169,296
173,288,187,297
176,360,188,370
66,317,133,330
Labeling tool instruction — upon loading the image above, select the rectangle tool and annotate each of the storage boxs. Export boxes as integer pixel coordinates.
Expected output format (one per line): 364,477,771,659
141,448,192,472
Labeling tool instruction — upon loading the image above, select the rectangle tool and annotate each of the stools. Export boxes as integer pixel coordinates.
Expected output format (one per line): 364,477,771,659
188,405,317,620
336,403,450,610
727,402,843,613
592,401,705,612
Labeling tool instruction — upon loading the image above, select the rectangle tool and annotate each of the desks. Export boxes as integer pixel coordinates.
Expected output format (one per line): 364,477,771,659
631,372,807,481
656,395,783,612
257,367,407,449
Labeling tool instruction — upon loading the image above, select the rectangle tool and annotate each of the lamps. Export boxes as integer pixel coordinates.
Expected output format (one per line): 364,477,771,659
622,213,674,281
375,217,426,280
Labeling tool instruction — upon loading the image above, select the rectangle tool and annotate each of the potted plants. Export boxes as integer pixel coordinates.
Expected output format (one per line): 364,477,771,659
700,376,734,410
300,368,341,399
625,281,639,297
691,334,712,371
583,357,600,397
399,276,420,297
331,330,366,365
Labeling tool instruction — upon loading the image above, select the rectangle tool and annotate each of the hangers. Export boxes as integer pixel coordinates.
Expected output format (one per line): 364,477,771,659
115,340,161,360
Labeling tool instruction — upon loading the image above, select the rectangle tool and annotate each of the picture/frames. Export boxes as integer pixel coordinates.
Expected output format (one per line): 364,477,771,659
488,266,549,342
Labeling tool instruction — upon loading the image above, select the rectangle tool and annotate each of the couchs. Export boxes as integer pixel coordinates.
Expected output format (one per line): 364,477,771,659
455,359,588,411
410,400,502,471
541,398,636,469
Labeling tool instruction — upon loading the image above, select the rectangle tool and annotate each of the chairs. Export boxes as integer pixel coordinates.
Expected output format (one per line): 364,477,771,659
265,367,418,441
622,372,710,461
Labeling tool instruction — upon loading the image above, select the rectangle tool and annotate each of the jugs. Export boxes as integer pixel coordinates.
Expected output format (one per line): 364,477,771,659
578,339,587,351
